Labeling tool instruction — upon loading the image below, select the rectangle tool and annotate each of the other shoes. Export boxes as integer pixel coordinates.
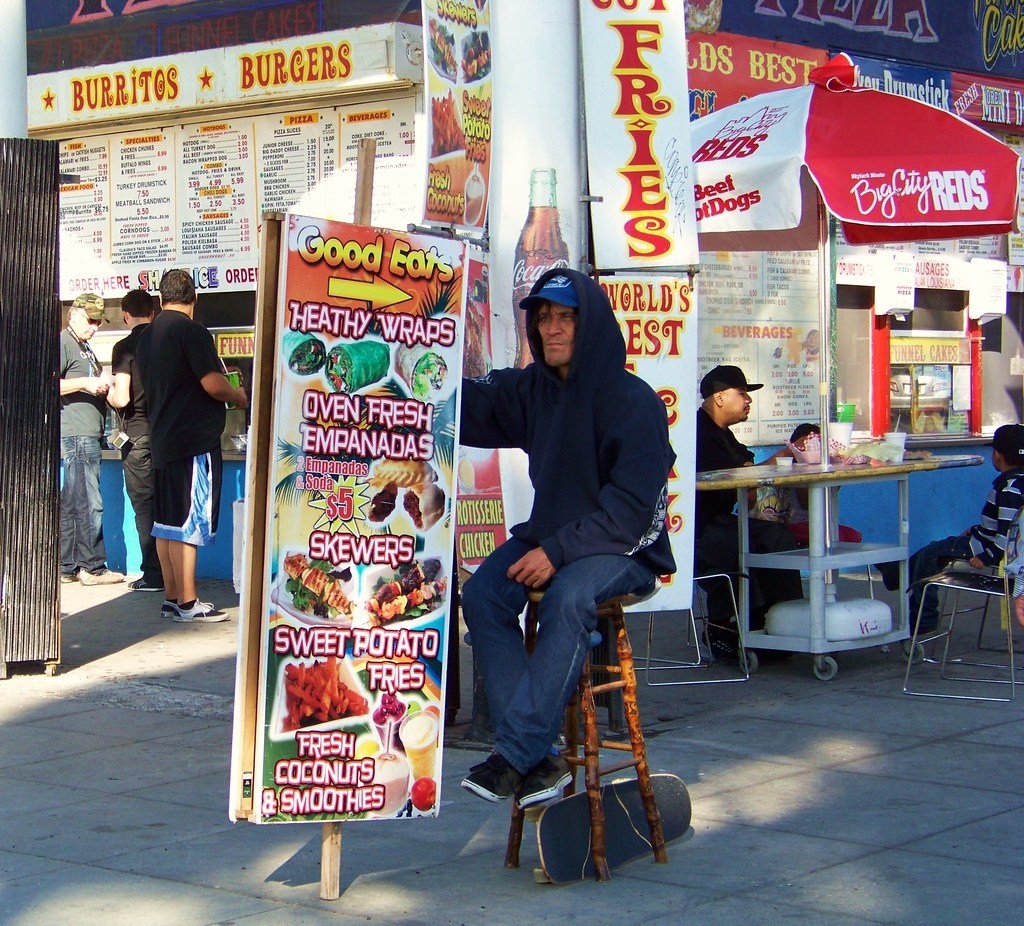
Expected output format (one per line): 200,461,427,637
900,627,937,642
873,562,896,591
701,626,740,658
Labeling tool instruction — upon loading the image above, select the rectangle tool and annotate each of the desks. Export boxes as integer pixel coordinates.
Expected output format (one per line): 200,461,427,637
696,455,986,679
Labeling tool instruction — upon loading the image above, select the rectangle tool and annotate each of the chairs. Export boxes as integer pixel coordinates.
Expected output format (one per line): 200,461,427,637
903,505,1024,702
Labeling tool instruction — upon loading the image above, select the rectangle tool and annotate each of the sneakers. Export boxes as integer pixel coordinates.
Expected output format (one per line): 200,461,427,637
172,598,229,622
460,749,524,804
79,567,124,586
127,578,164,592
515,750,573,811
61,572,80,583
160,600,177,617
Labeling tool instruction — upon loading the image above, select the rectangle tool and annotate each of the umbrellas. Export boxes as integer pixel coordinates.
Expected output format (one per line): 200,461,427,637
690,52,1023,583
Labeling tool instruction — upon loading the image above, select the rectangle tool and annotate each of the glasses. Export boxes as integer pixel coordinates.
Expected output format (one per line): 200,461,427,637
73,306,103,327
124,317,127,324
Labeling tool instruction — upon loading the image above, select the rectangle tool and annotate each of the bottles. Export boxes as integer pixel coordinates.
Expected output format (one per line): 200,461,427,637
512,168,571,368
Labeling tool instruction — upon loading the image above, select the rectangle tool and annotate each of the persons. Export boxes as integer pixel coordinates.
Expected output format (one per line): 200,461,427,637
788,423,863,549
459,269,677,808
136,268,247,623
60,294,125,585
105,289,164,593
874,423,1024,643
693,365,803,662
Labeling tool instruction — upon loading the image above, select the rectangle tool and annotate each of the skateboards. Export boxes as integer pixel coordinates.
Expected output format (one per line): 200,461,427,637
522,771,693,885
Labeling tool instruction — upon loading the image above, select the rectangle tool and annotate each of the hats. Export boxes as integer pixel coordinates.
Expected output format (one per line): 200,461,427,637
700,365,764,399
518,274,580,312
983,424,1024,456
72,292,110,324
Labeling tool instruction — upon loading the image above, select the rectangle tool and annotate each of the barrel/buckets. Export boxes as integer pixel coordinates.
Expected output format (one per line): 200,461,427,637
829,422,854,463
837,403,855,423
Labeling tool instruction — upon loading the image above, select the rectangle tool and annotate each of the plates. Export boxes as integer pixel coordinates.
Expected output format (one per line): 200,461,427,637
426,13,492,85
268,652,371,741
267,566,358,627
358,551,446,630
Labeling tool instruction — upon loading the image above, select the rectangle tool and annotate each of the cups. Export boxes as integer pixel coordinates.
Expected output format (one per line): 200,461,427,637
775,456,793,465
884,432,906,462
398,711,440,779
373,701,409,753
223,372,241,408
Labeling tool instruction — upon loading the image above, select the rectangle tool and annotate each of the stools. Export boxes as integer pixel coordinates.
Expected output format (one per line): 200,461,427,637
505,590,668,883
